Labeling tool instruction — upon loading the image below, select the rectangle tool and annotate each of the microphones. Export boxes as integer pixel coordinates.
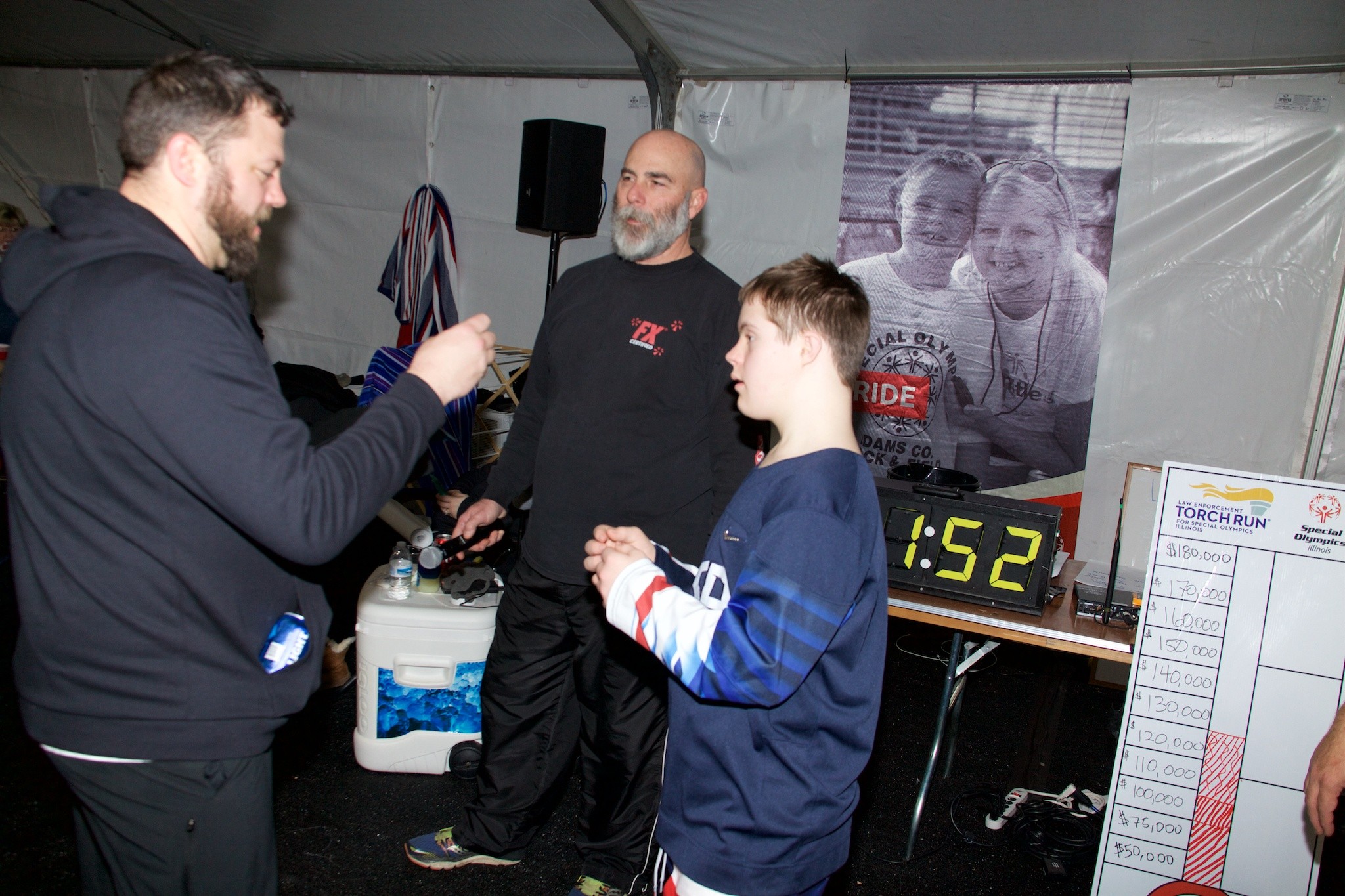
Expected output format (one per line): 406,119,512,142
417,516,513,569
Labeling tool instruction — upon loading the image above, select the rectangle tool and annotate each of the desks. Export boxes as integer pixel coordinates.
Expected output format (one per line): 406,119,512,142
888,559,1142,866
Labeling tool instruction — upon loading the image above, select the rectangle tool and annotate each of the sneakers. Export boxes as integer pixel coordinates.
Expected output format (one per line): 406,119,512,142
568,874,625,896
404,826,522,870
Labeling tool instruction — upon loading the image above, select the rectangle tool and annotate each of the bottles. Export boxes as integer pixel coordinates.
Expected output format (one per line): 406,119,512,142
389,541,413,600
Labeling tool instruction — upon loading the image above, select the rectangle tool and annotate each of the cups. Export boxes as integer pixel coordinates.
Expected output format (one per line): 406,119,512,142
393,545,424,587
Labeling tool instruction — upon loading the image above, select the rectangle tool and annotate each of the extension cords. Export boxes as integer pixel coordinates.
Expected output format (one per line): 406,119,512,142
985,787,1029,829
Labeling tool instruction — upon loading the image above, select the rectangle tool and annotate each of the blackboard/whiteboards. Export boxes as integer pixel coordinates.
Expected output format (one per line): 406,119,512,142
1092,461,1345,896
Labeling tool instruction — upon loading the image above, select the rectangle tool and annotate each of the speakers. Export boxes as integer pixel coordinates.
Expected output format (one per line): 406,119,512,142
515,118,606,235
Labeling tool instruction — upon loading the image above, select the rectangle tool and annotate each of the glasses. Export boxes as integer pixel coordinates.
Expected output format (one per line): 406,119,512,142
981,158,1071,227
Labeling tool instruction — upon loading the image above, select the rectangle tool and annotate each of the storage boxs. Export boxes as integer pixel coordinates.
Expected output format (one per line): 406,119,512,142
354,563,505,775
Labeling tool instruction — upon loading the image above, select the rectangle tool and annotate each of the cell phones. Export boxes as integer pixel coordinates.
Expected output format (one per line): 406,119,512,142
951,375,974,408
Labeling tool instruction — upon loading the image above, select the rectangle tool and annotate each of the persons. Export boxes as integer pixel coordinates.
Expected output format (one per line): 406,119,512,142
841,144,1004,489
956,152,1110,477
0,200,29,344
1300,702,1345,896
404,129,771,896
0,54,495,896
584,252,887,896
